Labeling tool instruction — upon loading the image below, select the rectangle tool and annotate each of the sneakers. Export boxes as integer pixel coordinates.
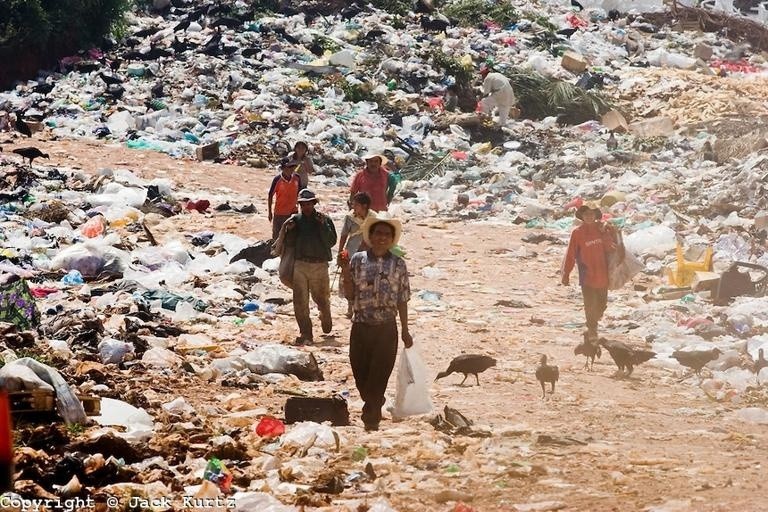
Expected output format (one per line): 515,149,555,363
321,321,332,333
296,335,314,345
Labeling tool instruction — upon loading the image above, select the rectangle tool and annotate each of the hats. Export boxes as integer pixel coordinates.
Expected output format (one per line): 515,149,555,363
575,201,602,220
297,189,318,204
363,211,403,249
477,68,488,75
361,150,389,167
280,156,297,168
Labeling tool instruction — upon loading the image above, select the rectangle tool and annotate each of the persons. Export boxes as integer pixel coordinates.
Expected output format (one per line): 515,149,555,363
267,140,413,434
474,65,515,126
561,200,618,340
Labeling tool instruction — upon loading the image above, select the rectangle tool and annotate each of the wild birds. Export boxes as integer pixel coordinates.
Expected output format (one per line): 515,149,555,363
598,338,656,375
435,352,496,387
75,0,301,102
754,348,768,376
417,13,458,36
534,354,558,395
555,26,581,41
340,6,364,23
365,29,386,42
668,346,726,376
310,33,325,59
572,331,603,372
32,80,57,96
14,109,31,136
12,146,49,166
